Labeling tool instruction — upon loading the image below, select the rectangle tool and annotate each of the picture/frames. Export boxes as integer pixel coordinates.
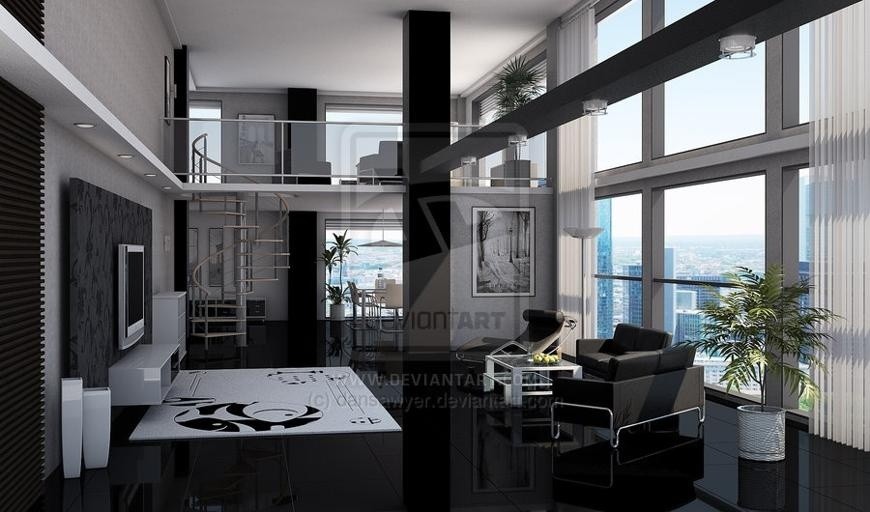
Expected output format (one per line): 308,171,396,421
473,206,535,297
163,55,172,125
237,112,277,164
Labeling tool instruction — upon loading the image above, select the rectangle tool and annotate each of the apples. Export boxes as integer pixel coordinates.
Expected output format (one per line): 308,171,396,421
543,357,549,363
548,356,555,363
534,355,543,362
552,355,559,361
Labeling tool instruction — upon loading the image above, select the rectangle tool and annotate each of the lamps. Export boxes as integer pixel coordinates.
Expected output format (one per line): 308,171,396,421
508,135,527,146
463,157,477,164
719,35,758,60
583,99,608,114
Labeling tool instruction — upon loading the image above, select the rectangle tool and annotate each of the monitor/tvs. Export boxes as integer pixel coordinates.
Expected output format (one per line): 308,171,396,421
114,243,147,351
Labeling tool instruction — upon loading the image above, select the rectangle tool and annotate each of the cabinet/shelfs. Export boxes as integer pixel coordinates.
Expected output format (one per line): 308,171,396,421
108,344,175,406
154,291,187,363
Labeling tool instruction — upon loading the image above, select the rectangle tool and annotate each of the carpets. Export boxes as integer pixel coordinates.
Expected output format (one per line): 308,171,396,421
128,365,404,443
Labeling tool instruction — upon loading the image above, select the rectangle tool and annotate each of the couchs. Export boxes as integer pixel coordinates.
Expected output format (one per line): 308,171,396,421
577,325,672,376
362,140,403,184
550,347,707,449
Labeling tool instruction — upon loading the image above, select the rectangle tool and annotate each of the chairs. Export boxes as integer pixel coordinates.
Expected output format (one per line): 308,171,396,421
347,280,373,321
454,308,578,364
378,284,408,332
352,282,379,328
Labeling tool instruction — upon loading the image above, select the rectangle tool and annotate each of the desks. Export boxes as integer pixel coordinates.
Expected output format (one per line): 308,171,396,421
364,288,397,326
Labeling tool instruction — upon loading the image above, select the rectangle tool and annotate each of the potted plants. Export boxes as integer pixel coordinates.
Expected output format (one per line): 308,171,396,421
313,228,359,322
664,265,847,461
487,54,551,119
